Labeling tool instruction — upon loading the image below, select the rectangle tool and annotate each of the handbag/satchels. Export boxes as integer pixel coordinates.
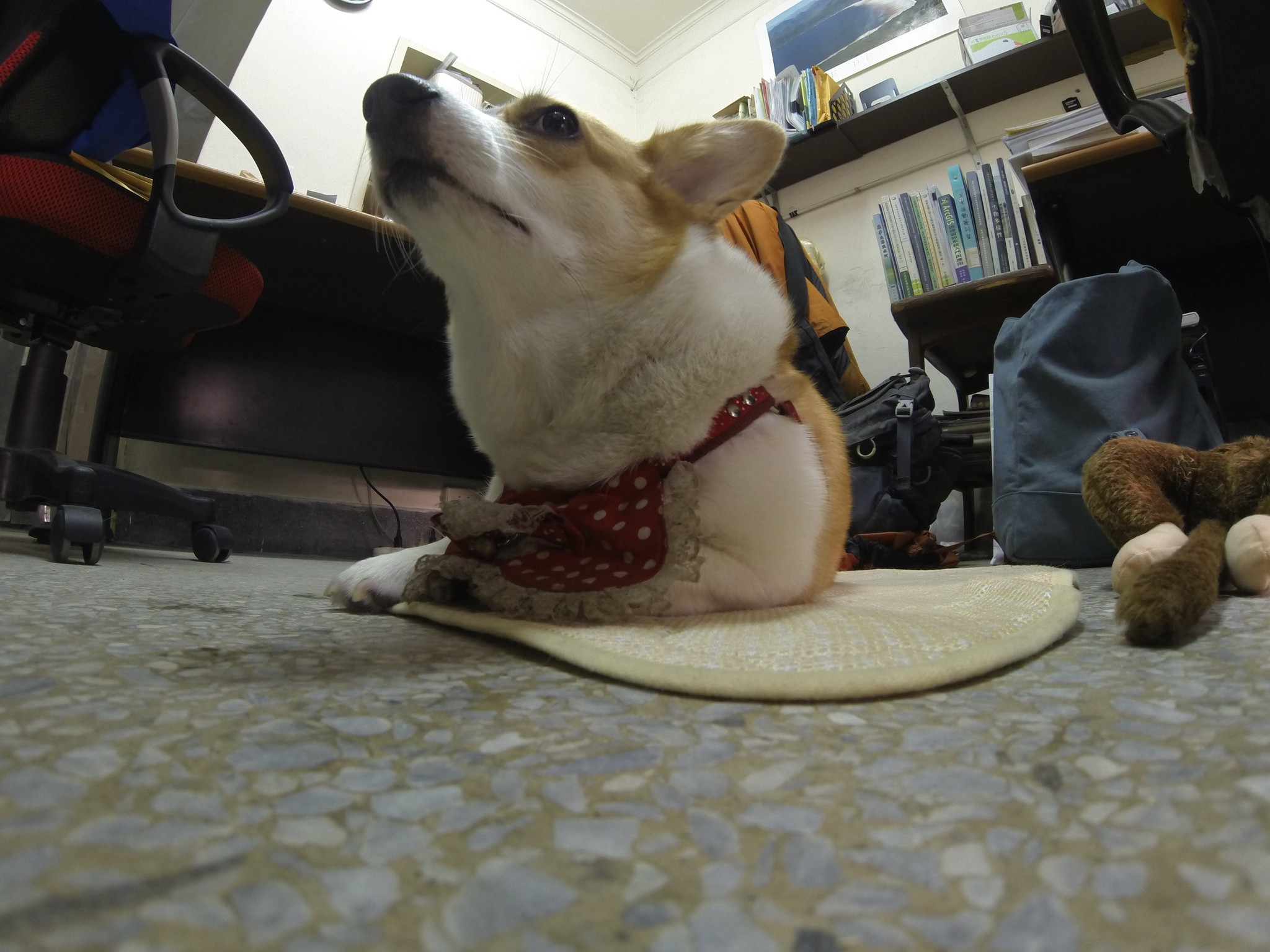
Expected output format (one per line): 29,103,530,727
833,365,975,535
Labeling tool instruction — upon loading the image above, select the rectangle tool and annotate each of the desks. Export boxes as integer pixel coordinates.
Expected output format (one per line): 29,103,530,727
758,3,1211,551
90,146,491,536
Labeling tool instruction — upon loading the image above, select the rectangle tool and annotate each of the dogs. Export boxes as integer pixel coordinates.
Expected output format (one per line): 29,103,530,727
326,73,855,622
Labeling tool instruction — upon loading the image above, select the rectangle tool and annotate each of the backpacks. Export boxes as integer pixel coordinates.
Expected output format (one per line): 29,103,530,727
991,259,1223,565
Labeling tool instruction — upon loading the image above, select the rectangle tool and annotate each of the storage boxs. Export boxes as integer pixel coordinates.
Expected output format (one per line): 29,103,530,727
957,1,1039,68
1043,0,1067,34
1039,14,1053,39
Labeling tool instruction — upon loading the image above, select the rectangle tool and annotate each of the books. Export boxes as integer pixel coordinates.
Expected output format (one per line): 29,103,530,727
873,158,1047,303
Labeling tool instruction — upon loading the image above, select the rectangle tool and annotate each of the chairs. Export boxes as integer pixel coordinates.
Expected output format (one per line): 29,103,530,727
0,0,297,564
1060,0,1270,443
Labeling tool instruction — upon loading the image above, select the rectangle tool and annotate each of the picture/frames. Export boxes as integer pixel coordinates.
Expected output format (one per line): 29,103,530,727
754,0,966,83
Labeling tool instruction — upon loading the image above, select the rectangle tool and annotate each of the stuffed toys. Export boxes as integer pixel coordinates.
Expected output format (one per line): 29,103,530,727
1081,435,1270,646
